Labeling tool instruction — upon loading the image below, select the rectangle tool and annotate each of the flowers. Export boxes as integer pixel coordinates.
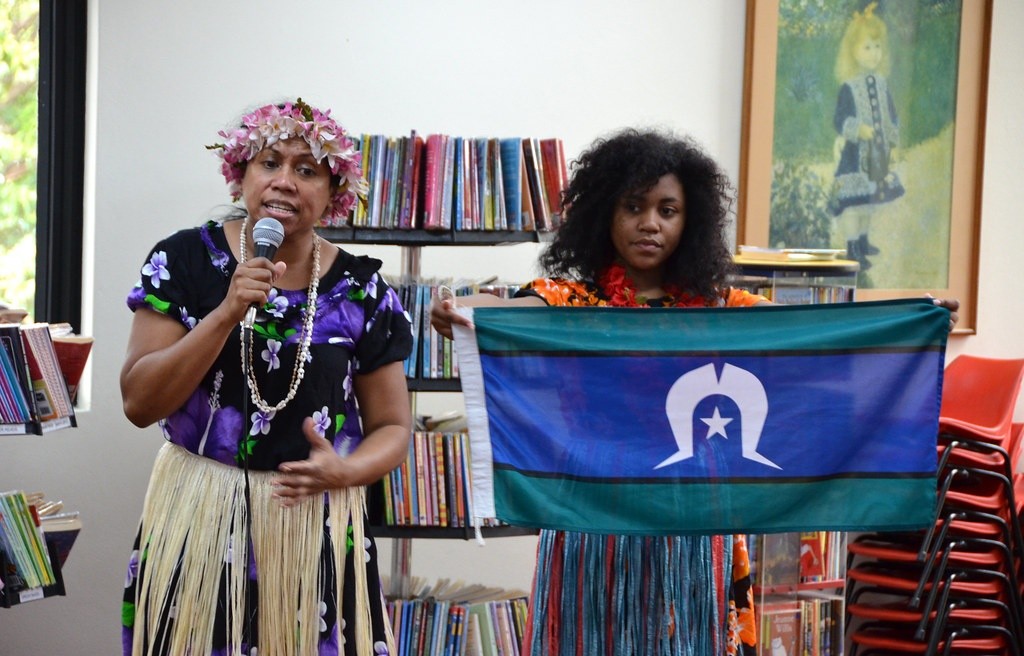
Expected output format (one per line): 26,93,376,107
205,98,370,223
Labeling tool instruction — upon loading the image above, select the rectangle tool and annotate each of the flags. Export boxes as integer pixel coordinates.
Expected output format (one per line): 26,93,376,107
453,298,949,533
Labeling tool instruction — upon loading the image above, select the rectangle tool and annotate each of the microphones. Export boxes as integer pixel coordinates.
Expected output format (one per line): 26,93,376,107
243,217,285,329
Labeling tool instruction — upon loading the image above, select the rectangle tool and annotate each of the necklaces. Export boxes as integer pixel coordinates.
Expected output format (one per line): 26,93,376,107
235,217,322,413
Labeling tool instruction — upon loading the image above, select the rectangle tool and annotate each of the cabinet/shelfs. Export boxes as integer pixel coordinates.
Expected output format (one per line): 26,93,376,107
0,416,78,608
315,226,540,544
729,254,861,656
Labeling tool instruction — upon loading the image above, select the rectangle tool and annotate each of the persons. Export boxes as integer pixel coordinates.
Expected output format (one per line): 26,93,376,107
120,97,416,656
428,128,961,656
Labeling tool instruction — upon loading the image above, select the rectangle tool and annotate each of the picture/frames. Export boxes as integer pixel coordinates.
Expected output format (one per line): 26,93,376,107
732,0,995,338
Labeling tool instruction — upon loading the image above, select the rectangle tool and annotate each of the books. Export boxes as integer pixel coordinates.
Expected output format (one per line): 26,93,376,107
744,533,842,584
311,129,575,233
738,590,843,656
364,415,511,528
0,489,84,589
725,278,852,304
383,574,532,656
0,321,96,426
383,280,527,379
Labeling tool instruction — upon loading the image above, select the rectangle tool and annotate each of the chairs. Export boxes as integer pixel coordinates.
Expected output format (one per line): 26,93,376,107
848,355,1024,656
